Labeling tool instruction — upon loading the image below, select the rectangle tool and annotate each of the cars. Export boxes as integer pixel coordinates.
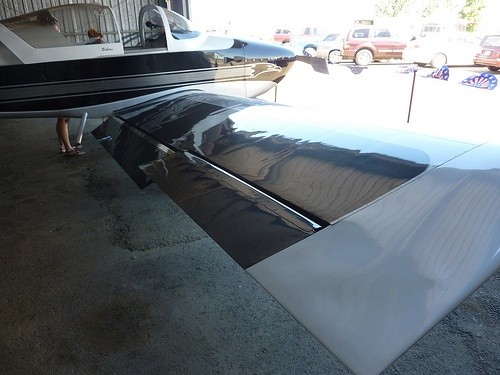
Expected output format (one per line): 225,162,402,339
317,29,344,64
473,34,500,71
401,31,484,68
273,28,317,57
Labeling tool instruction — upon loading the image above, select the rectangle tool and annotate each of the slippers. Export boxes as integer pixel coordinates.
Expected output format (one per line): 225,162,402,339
67,149,85,156
61,147,75,152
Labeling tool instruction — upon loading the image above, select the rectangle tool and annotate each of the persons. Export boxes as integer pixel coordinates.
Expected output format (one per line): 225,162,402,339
85,29,105,44
55,116,87,156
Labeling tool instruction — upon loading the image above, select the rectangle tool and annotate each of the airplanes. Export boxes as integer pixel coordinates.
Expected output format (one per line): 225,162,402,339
0,2,500,375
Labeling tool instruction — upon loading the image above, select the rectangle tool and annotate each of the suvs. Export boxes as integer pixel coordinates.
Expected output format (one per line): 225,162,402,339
341,25,407,66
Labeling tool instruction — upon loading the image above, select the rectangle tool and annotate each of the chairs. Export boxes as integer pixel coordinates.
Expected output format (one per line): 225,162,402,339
87,28,104,38
